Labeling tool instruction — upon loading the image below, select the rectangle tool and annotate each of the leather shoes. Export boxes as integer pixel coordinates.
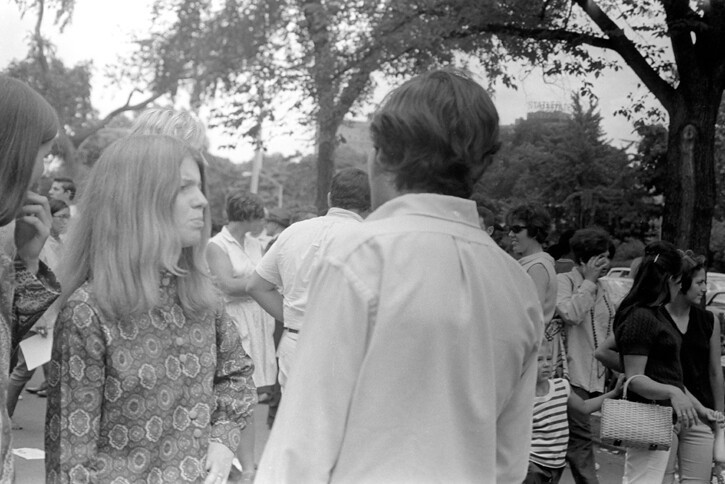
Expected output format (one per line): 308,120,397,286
36,388,47,397
25,387,42,394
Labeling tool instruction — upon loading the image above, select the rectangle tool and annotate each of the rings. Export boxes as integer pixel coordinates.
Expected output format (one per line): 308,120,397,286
595,262,600,266
219,473,223,479
682,412,686,416
38,330,41,333
40,329,44,334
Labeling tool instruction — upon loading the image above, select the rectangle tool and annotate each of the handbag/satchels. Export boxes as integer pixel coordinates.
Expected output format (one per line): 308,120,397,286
600,372,672,451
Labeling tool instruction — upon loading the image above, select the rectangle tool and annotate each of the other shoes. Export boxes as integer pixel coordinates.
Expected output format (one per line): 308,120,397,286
9,417,23,430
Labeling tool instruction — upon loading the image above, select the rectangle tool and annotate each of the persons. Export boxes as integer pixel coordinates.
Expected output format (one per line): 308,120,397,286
253,71,544,484
468,194,725,484
0,72,372,484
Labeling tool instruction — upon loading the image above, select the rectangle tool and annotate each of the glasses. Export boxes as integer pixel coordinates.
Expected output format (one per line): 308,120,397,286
506,225,526,233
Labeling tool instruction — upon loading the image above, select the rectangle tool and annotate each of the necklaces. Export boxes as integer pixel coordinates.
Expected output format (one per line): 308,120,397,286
670,304,689,322
590,292,612,379
